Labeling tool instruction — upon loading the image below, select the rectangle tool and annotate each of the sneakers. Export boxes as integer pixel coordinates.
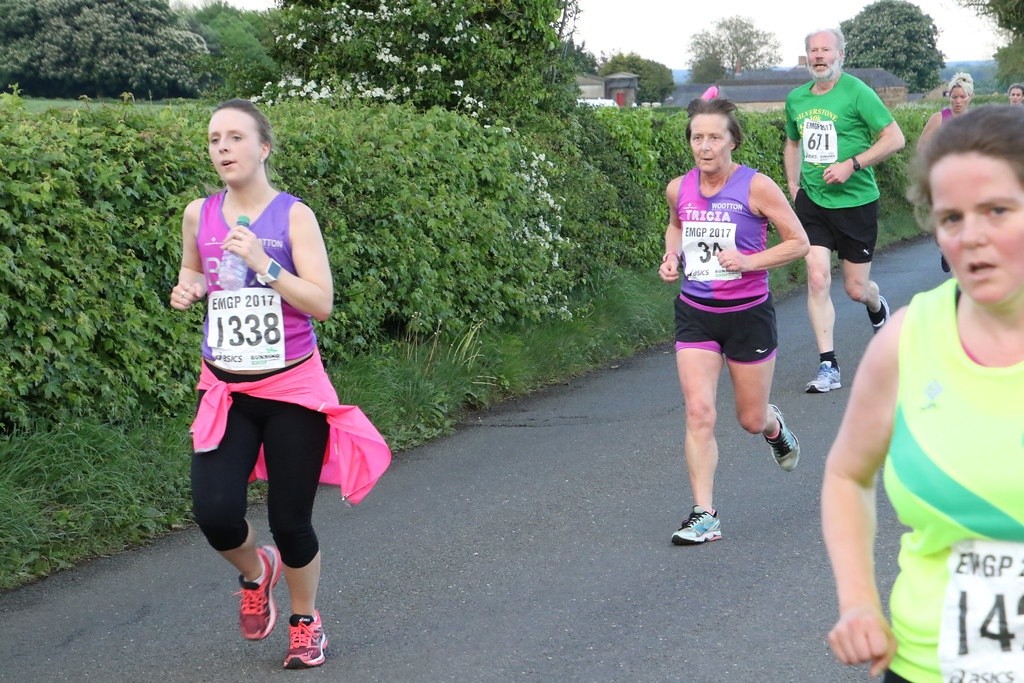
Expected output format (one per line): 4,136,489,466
865,296,890,334
804,356,842,392
763,404,801,472
672,506,722,544
231,545,283,640
283,609,328,669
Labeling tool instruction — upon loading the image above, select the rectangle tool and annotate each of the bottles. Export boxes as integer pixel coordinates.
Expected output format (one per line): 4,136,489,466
217,215,250,292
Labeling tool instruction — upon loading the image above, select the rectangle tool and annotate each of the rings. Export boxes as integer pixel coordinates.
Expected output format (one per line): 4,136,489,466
729,261,731,265
180,291,186,298
185,284,190,291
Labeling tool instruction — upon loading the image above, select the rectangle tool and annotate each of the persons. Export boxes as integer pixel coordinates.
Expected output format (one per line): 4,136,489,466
784,28,905,393
170,99,392,670
916,72,974,272
658,97,810,544
820,102,1024,683
1007,84,1024,105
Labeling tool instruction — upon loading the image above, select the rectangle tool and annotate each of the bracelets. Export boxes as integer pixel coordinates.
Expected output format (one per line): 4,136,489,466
663,251,680,262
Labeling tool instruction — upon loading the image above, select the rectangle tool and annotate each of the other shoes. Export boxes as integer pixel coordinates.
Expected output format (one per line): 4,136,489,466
941,256,951,273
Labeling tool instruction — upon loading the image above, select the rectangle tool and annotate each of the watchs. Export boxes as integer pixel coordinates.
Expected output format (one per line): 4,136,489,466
256,258,281,285
852,157,861,172
